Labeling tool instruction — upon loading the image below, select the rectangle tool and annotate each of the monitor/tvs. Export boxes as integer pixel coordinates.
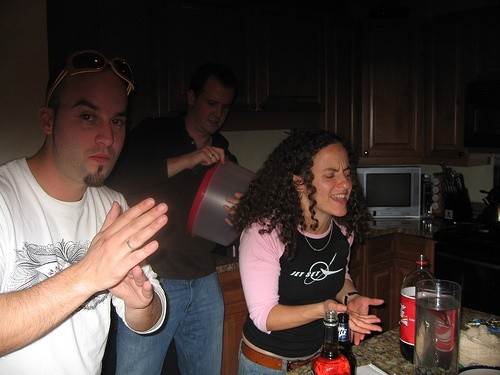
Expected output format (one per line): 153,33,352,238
356,166,421,219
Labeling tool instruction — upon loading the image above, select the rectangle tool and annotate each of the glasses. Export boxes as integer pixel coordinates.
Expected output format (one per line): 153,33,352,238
45,49,135,106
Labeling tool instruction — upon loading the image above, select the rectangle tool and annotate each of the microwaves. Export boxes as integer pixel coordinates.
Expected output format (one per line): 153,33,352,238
354,167,421,221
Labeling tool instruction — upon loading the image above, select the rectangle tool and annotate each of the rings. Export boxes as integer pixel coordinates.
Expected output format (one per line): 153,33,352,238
125,238,135,251
209,153,215,159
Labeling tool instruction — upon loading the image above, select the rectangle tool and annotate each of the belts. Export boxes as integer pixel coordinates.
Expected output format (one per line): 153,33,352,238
241,341,323,372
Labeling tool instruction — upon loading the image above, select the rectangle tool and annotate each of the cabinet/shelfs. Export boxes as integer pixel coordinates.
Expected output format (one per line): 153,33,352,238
46,2,498,375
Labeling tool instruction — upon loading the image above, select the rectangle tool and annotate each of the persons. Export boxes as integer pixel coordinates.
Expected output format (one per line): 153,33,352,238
230,125,385,375
0,44,169,374
104,59,245,374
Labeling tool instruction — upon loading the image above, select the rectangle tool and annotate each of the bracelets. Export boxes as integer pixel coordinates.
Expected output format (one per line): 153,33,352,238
343,291,360,305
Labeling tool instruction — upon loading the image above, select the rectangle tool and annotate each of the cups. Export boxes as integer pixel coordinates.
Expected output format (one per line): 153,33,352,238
412,279,462,375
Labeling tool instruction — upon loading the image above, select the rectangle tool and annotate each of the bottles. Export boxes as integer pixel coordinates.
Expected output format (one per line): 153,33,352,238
399,255,436,364
311,310,351,375
337,312,357,375
431,176,444,217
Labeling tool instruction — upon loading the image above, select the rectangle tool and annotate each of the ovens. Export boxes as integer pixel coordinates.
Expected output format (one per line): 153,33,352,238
434,241,500,318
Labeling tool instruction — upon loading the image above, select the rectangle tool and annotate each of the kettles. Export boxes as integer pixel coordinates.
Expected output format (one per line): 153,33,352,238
481,198,500,223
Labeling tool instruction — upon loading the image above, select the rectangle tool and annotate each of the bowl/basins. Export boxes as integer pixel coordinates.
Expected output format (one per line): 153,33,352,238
187,155,258,247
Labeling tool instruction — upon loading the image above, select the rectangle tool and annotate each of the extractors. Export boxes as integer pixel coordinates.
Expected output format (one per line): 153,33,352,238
464,79,500,154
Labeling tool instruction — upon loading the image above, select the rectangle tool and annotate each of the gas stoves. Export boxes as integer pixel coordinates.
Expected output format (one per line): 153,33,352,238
433,220,500,254
